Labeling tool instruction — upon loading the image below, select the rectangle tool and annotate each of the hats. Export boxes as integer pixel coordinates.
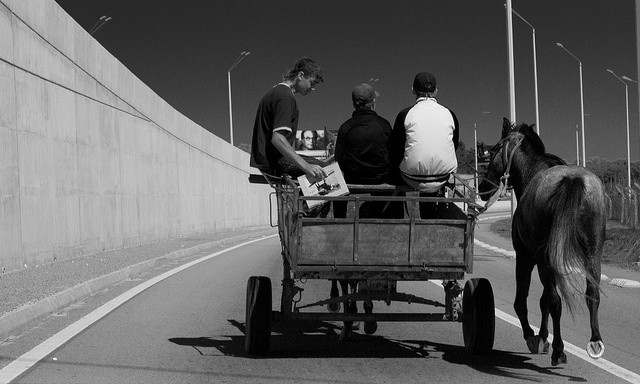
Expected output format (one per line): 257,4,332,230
352,83,380,101
415,72,436,92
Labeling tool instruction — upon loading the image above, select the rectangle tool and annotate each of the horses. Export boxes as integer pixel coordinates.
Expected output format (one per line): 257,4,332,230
478,116,610,367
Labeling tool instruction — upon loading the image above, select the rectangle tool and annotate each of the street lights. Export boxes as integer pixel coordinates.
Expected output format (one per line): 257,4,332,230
90,15,111,37
556,41,586,168
606,68,631,200
227,50,251,144
473,110,491,198
621,75,638,84
504,2,540,136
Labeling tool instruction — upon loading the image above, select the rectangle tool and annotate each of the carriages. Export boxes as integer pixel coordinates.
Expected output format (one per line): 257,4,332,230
242,171,495,356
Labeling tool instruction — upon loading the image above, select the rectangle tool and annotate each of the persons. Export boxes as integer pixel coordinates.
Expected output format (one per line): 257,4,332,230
250,57,326,184
333,83,394,218
299,130,323,150
393,71,459,217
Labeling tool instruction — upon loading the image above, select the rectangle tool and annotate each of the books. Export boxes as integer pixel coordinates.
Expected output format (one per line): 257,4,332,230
297,161,351,210
